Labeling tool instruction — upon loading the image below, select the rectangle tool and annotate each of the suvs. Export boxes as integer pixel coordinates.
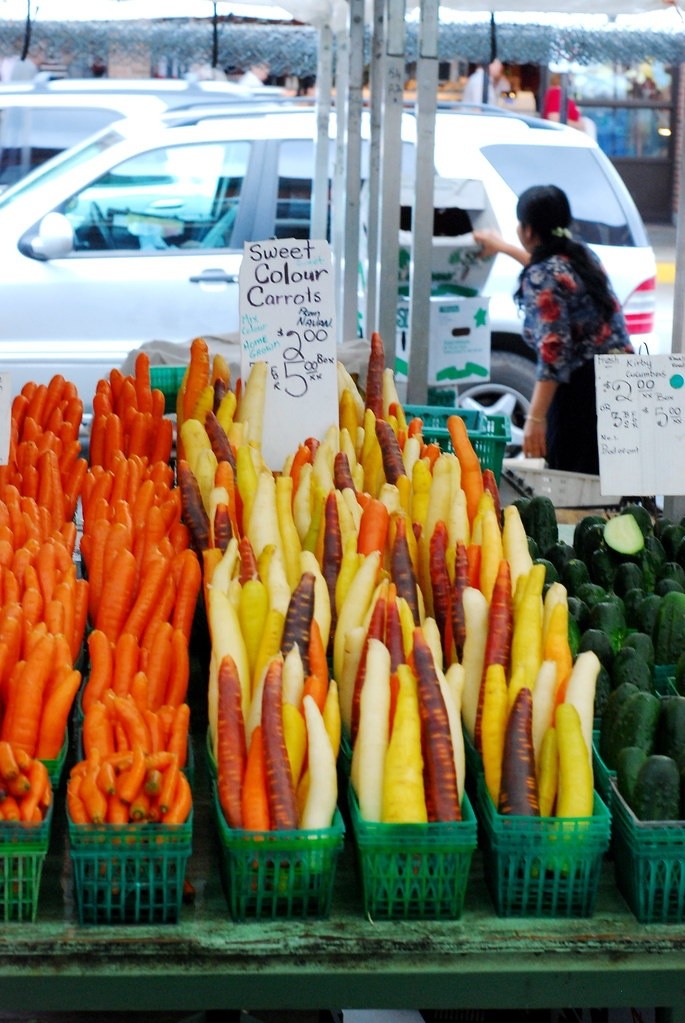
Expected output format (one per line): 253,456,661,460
0,69,660,461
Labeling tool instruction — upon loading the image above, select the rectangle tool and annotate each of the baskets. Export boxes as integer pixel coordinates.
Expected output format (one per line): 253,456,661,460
341,728,477,918
0,586,87,928
461,725,611,916
589,664,685,923
398,404,512,490
206,725,345,921
64,548,194,922
132,364,196,395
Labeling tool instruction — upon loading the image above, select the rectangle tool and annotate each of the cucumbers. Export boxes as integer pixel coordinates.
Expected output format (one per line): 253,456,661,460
511,495,685,822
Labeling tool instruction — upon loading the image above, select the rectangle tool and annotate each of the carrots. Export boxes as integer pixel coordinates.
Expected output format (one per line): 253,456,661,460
0,332,600,890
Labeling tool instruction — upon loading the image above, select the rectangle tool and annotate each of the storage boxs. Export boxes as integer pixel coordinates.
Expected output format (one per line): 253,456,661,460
405,406,513,487
388,242,500,388
1,560,685,925
151,365,192,407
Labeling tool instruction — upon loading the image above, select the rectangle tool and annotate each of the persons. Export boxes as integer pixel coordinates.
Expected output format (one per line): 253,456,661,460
472,183,639,479
0,31,580,129
